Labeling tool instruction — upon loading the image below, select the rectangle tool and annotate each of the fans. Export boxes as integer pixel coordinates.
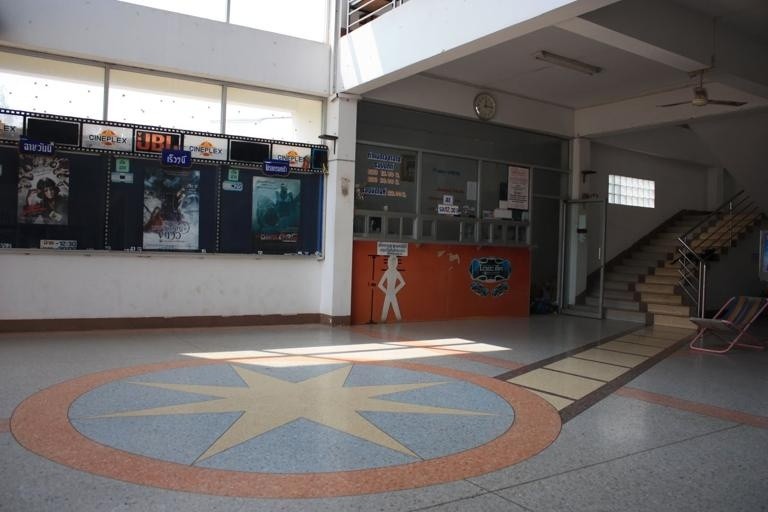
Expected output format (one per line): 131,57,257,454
653,69,747,108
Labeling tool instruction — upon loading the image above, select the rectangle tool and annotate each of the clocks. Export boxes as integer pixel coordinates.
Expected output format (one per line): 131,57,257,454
474,93,497,120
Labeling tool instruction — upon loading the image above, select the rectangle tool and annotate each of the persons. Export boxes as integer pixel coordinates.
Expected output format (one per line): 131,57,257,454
25,177,69,217
467,213,490,242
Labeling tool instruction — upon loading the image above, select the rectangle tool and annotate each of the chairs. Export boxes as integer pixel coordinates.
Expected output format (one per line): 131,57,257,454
688,294,768,354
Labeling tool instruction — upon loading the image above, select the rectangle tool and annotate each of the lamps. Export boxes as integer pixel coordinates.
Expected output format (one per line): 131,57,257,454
532,50,605,78
